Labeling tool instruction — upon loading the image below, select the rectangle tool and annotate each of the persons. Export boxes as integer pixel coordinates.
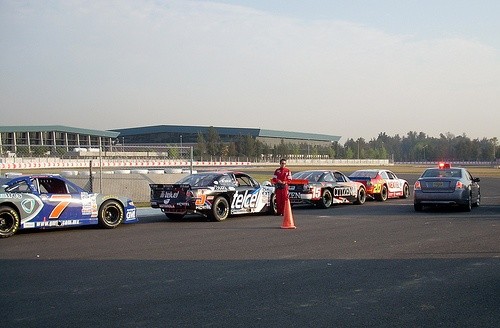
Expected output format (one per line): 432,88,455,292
273,159,292,216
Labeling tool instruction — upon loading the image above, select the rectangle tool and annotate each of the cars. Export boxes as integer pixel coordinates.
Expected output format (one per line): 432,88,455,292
413,162,482,213
0,174,141,239
287,169,367,210
348,169,409,201
148,170,279,222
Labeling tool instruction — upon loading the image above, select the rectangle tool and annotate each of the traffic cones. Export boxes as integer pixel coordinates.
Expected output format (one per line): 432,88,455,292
279,198,297,229
